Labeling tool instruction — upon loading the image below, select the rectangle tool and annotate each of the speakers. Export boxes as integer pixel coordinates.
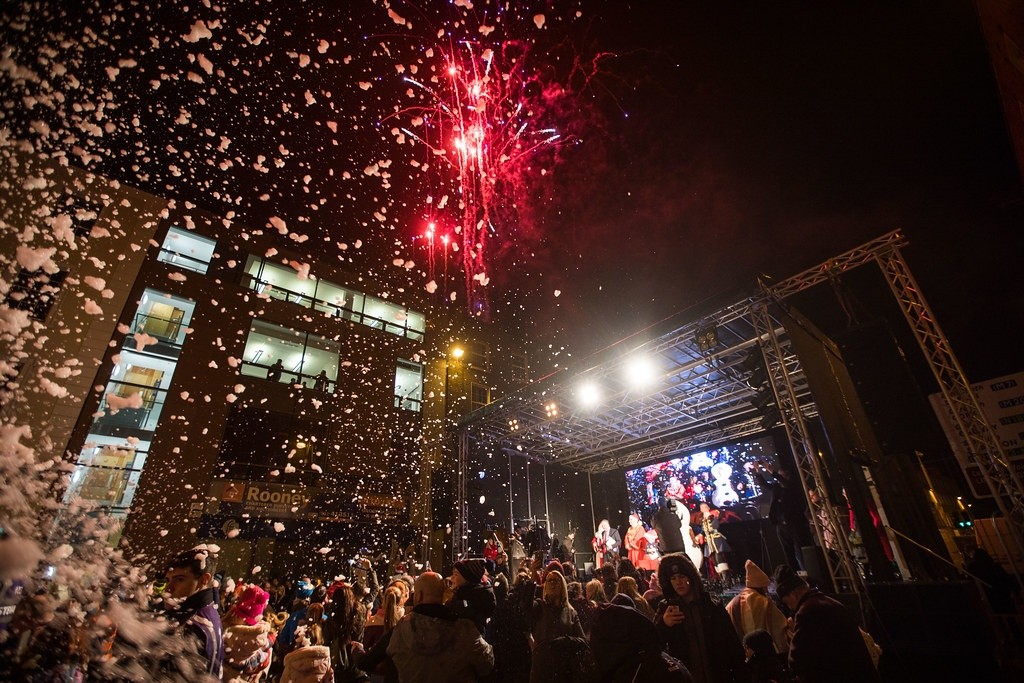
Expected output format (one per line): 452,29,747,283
802,545,841,594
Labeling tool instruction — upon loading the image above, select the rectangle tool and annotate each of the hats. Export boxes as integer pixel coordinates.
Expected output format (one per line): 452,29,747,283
233,585,270,625
453,559,486,582
744,559,770,588
773,564,807,600
667,499,677,506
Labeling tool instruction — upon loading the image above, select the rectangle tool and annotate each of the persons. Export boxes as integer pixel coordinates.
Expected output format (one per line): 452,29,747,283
289,377,307,389
0,497,1024,683
312,370,329,393
646,475,752,512
265,358,284,382
